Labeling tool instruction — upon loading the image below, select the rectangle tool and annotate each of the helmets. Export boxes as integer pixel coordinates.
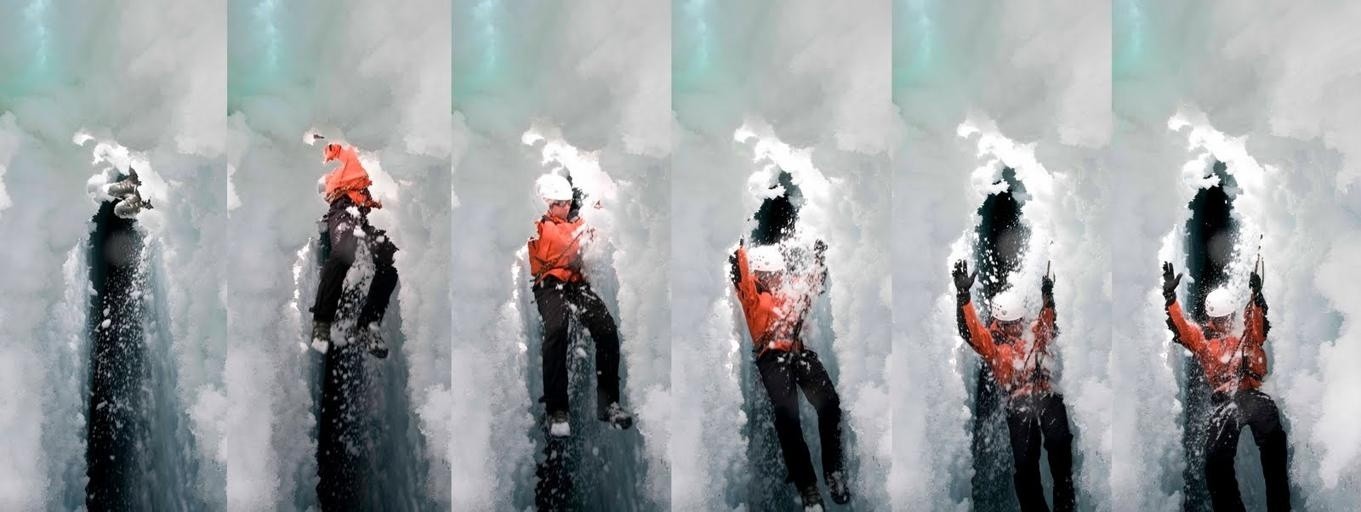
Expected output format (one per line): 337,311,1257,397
540,175,573,200
1205,289,1237,318
751,245,785,273
992,292,1025,322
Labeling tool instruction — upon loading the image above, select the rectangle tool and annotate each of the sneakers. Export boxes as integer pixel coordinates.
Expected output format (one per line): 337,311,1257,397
545,410,569,437
597,401,632,429
799,485,824,512
357,327,389,357
823,471,849,505
312,321,330,343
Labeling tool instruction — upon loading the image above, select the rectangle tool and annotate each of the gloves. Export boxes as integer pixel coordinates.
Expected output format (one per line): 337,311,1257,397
1249,272,1262,307
1042,276,1055,307
1162,261,1183,306
952,259,979,296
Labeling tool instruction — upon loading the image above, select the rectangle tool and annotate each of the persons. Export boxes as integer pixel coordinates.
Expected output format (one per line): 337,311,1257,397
1159,259,1292,511
526,172,634,439
728,235,853,510
951,256,1077,509
310,131,398,359
